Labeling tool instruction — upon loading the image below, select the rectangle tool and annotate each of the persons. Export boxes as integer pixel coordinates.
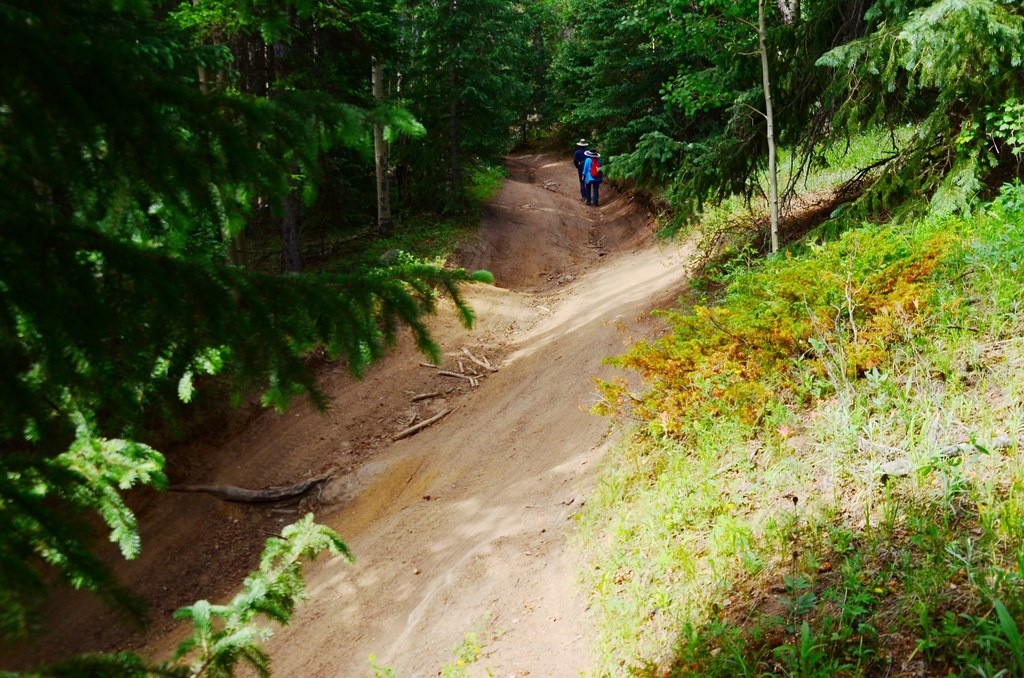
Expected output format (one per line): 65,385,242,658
573,139,604,207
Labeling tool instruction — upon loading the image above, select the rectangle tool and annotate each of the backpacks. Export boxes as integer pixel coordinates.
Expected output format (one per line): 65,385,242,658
591,158,603,178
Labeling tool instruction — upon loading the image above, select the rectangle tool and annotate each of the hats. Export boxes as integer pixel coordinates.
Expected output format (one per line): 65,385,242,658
584,148,600,157
577,139,588,146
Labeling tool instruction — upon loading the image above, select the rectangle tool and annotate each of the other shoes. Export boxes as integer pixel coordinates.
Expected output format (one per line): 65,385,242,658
593,204,599,207
581,197,585,201
584,199,591,204
